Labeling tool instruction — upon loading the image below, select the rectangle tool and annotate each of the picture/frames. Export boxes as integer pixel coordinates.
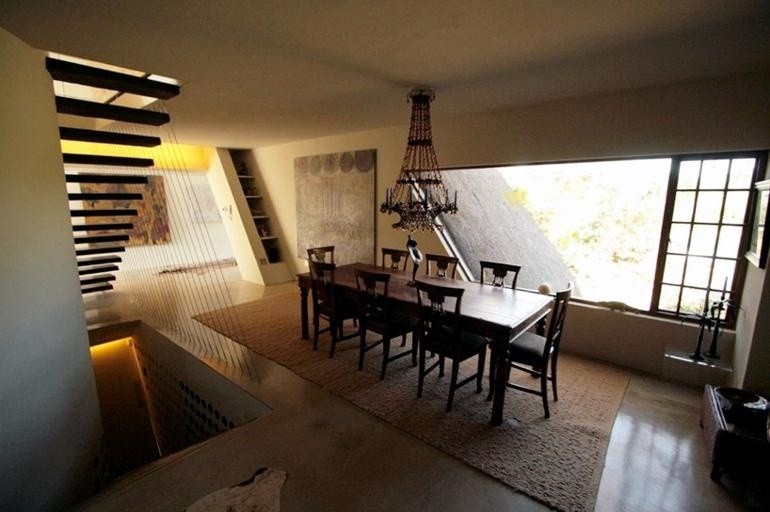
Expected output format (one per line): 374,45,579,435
741,179,770,269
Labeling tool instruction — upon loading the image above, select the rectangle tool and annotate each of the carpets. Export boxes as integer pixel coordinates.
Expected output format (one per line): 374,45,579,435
189,289,633,512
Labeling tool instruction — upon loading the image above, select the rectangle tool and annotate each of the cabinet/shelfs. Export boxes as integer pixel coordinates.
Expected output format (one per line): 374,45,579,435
699,384,769,482
202,147,295,286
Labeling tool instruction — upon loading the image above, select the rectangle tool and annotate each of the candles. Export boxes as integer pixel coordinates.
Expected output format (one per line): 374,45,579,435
705,280,710,309
722,276,728,298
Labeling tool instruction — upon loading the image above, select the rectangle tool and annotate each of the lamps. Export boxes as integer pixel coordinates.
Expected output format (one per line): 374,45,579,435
380,87,457,233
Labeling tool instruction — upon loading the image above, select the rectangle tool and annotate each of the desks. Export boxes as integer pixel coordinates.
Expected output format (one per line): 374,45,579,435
295,261,554,427
659,338,734,409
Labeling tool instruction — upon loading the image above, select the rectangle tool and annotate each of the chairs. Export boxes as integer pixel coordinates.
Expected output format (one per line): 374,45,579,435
381,249,410,271
413,280,486,411
488,284,571,417
400,254,461,358
479,262,522,290
353,268,418,380
308,261,361,357
307,246,356,335
